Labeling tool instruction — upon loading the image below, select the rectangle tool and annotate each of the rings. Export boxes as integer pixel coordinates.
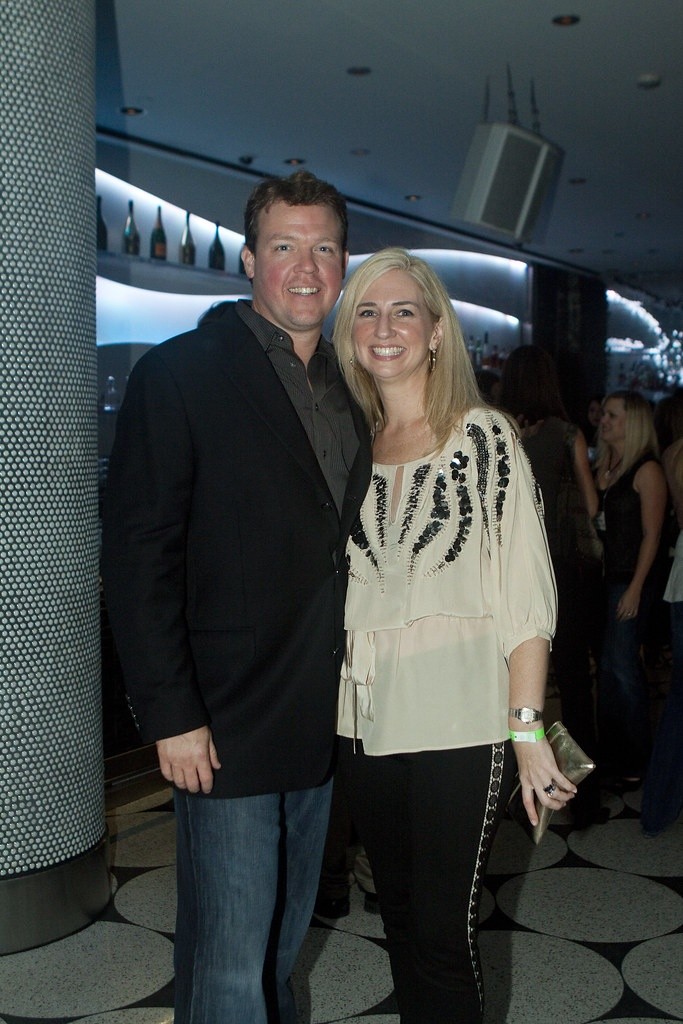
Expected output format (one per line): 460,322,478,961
543,783,556,797
628,608,633,616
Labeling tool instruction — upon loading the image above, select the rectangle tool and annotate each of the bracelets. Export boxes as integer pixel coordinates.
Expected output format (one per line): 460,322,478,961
508,727,545,743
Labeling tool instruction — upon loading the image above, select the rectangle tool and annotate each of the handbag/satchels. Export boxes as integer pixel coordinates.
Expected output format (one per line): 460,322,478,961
504,722,597,844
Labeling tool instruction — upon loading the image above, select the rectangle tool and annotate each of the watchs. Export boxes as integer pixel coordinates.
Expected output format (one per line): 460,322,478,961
509,706,544,725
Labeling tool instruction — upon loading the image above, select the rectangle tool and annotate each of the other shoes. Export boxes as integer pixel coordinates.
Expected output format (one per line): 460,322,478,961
317,894,350,919
573,807,611,830
620,778,643,792
364,893,381,913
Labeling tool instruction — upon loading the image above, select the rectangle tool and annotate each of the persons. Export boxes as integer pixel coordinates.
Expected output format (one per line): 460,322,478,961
501,344,683,838
330,247,577,1024
96,171,374,1024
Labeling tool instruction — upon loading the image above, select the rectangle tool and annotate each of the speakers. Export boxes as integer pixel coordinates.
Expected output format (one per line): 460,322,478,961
451,118,551,239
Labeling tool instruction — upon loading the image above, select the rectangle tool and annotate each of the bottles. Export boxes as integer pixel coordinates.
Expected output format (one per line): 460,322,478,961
123,200,140,256
177,209,196,266
463,332,514,379
97,195,107,252
207,221,225,271
149,207,167,260
